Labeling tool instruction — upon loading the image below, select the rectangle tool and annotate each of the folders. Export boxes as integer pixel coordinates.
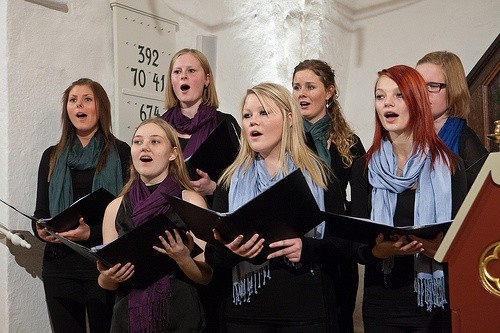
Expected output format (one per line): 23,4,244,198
170,115,240,189
159,167,324,266
322,209,455,243
0,186,115,236
45,213,205,291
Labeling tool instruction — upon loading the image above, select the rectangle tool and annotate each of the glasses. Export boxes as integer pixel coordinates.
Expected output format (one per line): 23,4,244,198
425,81,448,93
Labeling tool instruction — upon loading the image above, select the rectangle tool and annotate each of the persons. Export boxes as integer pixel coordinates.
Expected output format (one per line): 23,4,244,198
159,48,243,209
32,77,133,333
348,64,467,333
98,120,208,333
204,83,346,333
293,59,372,333
415,52,488,190
